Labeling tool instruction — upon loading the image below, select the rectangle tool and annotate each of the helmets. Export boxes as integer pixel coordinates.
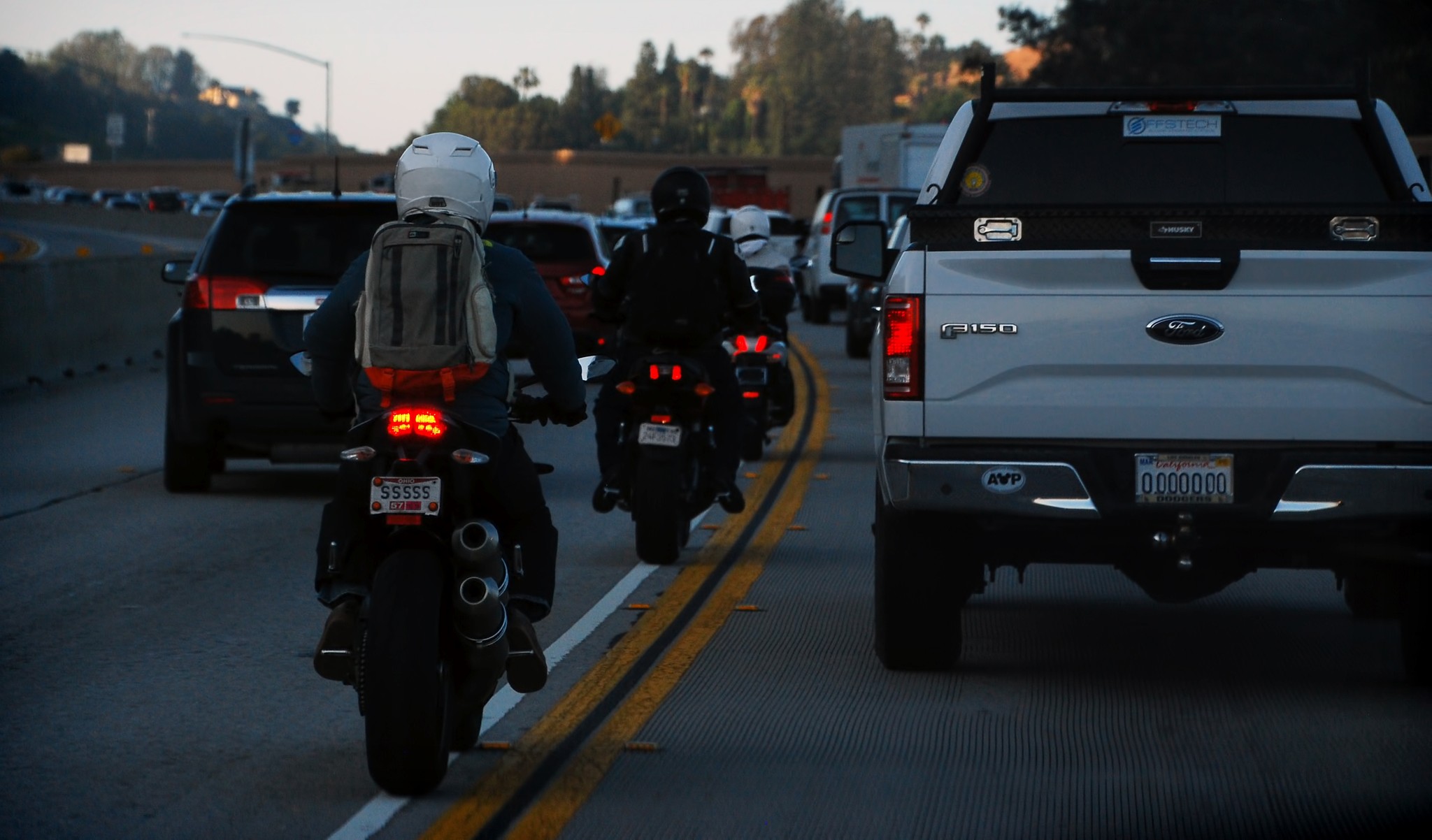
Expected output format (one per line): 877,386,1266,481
651,165,710,226
395,132,497,235
730,205,770,240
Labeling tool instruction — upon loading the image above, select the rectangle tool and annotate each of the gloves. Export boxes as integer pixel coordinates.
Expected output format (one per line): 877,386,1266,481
537,394,586,426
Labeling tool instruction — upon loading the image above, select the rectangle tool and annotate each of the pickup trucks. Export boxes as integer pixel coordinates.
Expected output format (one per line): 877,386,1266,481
791,187,922,328
828,63,1432,696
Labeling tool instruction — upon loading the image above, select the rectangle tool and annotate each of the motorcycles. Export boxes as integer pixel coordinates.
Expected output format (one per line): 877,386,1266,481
592,263,776,567
333,351,557,799
721,255,820,461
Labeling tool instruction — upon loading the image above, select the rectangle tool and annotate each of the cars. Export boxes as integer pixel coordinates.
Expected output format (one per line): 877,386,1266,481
0,178,232,219
164,179,399,498
484,208,613,356
489,194,808,282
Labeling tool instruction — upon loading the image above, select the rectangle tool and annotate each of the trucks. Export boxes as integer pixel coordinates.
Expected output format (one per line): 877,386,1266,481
831,119,950,216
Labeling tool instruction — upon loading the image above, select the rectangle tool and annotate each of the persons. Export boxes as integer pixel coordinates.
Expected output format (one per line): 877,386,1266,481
730,204,790,380
592,166,759,516
301,132,588,694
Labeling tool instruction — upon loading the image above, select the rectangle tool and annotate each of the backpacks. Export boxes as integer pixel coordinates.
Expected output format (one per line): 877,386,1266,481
354,208,497,393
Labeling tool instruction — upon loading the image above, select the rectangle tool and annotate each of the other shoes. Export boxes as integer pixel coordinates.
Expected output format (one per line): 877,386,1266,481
315,605,361,679
506,612,547,693
593,482,621,512
751,431,766,461
717,481,746,512
